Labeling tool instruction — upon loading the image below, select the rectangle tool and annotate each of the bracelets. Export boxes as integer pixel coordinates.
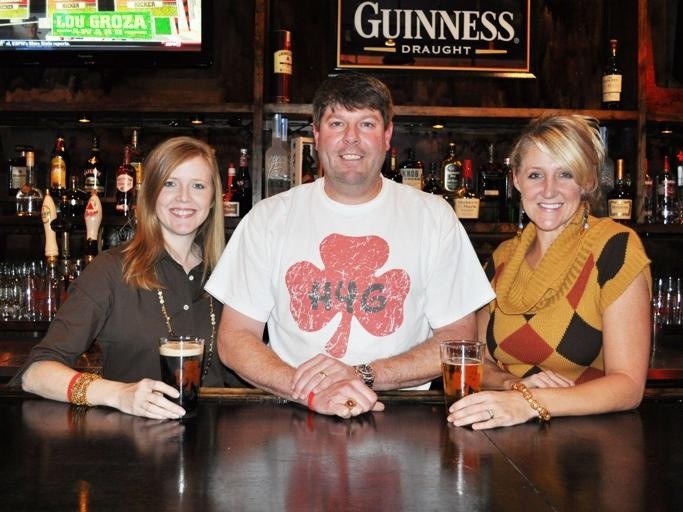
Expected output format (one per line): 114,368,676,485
71,405,88,433
67,371,84,403
67,404,75,431
306,391,316,413
511,380,553,423
70,371,100,406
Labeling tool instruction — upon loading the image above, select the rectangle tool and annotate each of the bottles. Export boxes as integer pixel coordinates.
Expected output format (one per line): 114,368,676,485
598,38,627,109
1,125,253,325
388,141,520,225
597,128,682,227
261,114,315,198
269,25,294,104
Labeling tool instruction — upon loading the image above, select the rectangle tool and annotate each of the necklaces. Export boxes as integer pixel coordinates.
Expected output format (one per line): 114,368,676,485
155,271,217,386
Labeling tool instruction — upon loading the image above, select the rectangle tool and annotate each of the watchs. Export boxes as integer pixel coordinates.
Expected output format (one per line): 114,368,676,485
356,362,375,390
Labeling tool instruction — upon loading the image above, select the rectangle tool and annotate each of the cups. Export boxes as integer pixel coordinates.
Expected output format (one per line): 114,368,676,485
439,340,486,418
158,336,206,419
653,276,680,329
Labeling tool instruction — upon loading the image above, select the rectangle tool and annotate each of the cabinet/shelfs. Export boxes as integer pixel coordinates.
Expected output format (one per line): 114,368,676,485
0,0,683,381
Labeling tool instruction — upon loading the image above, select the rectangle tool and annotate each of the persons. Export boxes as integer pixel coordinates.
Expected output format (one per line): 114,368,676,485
445,113,654,430
199,72,497,419
208,400,491,511
13,135,254,421
16,395,190,465
445,410,647,511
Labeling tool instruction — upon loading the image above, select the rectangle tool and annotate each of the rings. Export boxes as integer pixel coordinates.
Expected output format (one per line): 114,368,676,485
320,371,328,380
342,395,357,411
486,408,497,421
